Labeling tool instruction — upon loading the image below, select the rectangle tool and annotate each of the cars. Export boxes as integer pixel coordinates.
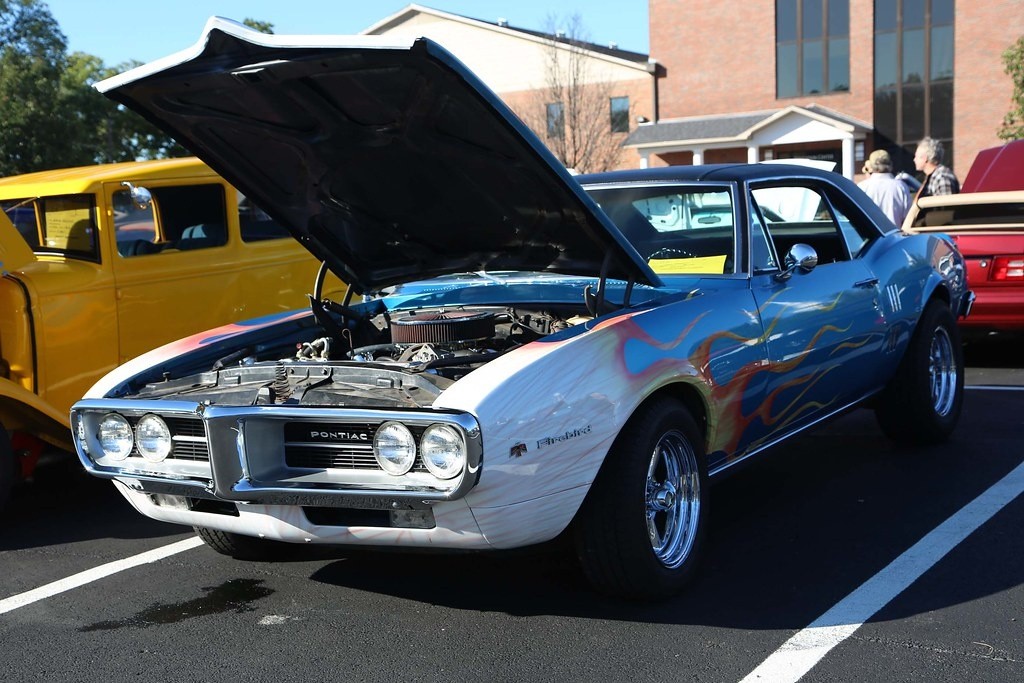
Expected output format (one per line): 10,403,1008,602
67,18,976,586
910,140,1024,336
632,158,836,231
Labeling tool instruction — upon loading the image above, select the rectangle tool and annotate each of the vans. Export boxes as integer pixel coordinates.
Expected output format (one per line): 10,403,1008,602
0,156,365,532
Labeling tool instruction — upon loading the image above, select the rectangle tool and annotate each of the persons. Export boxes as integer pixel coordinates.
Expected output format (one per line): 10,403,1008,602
913,135,960,199
856,149,913,227
